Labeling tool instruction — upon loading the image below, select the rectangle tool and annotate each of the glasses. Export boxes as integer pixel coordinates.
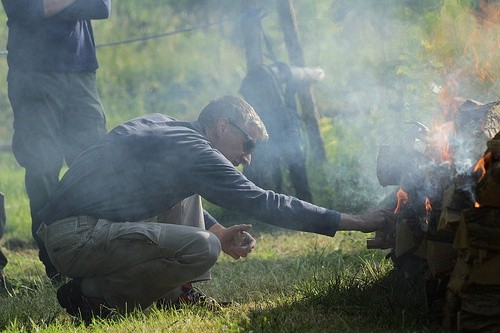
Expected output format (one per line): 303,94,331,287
228,119,257,155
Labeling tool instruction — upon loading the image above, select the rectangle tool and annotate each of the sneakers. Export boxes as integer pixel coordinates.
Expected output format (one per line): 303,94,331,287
56,280,110,326
155,280,228,315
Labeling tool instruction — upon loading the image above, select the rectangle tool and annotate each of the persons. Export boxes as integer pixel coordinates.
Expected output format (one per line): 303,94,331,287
35,94,403,329
1,1,112,290
